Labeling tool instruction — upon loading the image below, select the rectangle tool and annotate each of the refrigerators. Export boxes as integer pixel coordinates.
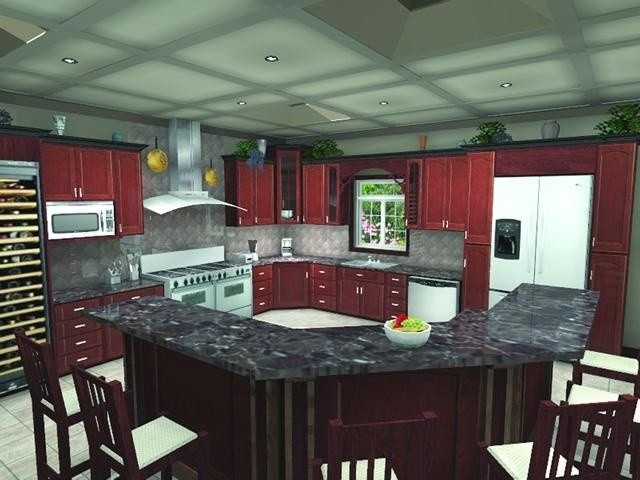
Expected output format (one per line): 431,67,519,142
488,175,594,313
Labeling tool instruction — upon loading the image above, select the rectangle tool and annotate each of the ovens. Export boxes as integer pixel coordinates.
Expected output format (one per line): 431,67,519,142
172,274,253,319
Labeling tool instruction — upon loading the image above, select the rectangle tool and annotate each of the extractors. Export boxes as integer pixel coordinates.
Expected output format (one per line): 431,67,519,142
144,118,248,215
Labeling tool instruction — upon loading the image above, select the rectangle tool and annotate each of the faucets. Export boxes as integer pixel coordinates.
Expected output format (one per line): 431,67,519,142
368,254,374,263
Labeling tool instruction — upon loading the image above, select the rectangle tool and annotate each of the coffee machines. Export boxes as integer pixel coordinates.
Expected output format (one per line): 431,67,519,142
280,238,294,256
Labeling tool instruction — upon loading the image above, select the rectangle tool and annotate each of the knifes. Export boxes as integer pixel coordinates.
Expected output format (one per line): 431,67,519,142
107,259,123,276
126,253,141,270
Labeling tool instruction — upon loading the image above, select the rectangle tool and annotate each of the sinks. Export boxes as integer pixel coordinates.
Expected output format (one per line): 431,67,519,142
345,258,395,269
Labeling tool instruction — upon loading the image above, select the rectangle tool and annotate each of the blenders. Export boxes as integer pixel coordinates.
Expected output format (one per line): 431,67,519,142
247,239,259,261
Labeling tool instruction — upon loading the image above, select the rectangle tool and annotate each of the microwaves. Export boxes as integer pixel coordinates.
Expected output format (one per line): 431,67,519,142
46,200,114,243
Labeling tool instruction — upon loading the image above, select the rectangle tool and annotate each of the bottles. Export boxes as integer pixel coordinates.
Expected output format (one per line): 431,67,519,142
0,178,41,378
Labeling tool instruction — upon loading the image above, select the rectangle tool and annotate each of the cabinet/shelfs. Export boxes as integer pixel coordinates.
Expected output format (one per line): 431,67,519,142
337,267,386,320
0,124,150,246
49,285,164,378
385,273,408,322
224,157,472,232
309,264,337,312
273,263,308,308
459,142,637,356
252,264,273,314
0,160,52,396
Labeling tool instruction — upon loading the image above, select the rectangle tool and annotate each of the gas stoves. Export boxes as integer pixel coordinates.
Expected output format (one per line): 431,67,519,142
148,259,247,278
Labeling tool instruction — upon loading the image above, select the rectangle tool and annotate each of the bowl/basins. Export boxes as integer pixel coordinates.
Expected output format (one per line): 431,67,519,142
384,319,432,348
281,209,293,218
